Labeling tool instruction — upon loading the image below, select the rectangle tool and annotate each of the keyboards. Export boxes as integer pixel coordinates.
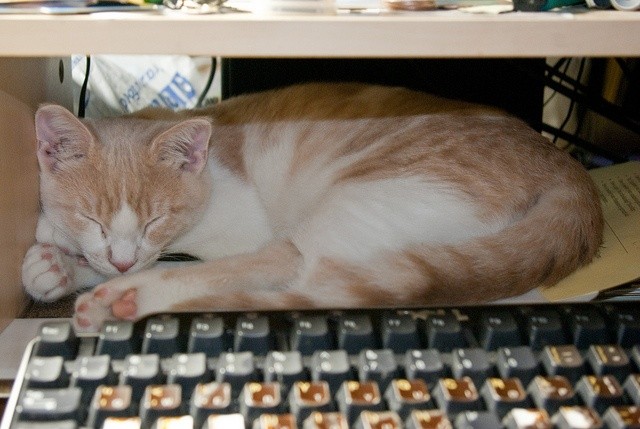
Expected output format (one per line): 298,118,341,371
0,296,640,428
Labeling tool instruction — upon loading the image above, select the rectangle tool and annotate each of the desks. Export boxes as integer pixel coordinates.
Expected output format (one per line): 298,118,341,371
0,5,640,344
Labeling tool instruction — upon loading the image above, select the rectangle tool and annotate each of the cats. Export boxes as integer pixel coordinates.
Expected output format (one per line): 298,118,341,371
20,78,609,339
